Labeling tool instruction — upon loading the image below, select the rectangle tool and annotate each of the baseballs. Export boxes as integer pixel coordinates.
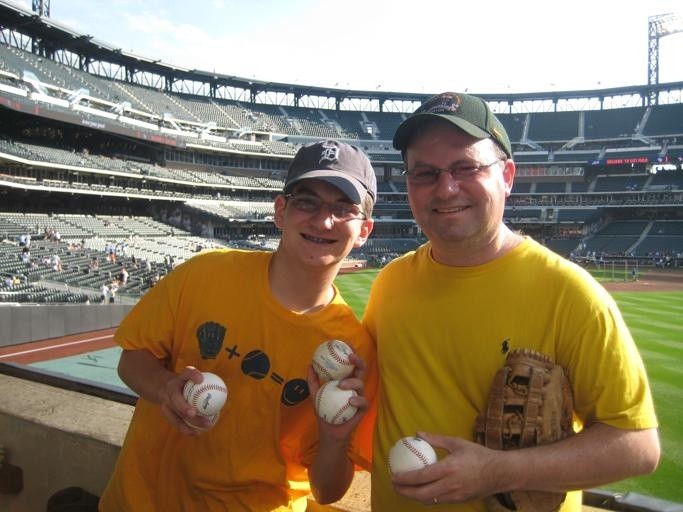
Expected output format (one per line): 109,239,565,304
311,338,359,426
389,437,438,481
179,371,229,433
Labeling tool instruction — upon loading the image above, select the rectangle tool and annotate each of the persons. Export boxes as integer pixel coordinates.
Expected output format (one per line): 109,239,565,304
354,89,662,511
94,138,379,512
504,209,682,271
0,209,224,308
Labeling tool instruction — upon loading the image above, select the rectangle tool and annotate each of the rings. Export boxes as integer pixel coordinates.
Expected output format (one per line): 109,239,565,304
431,494,439,505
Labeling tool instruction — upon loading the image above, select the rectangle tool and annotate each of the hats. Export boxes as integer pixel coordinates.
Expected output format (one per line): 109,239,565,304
283,138,377,206
393,92,511,155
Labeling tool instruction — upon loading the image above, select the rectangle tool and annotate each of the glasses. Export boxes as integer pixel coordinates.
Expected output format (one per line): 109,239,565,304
283,187,368,222
400,158,505,187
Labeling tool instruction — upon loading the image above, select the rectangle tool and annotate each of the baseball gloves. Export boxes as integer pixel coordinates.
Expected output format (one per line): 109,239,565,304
474,347,576,512
